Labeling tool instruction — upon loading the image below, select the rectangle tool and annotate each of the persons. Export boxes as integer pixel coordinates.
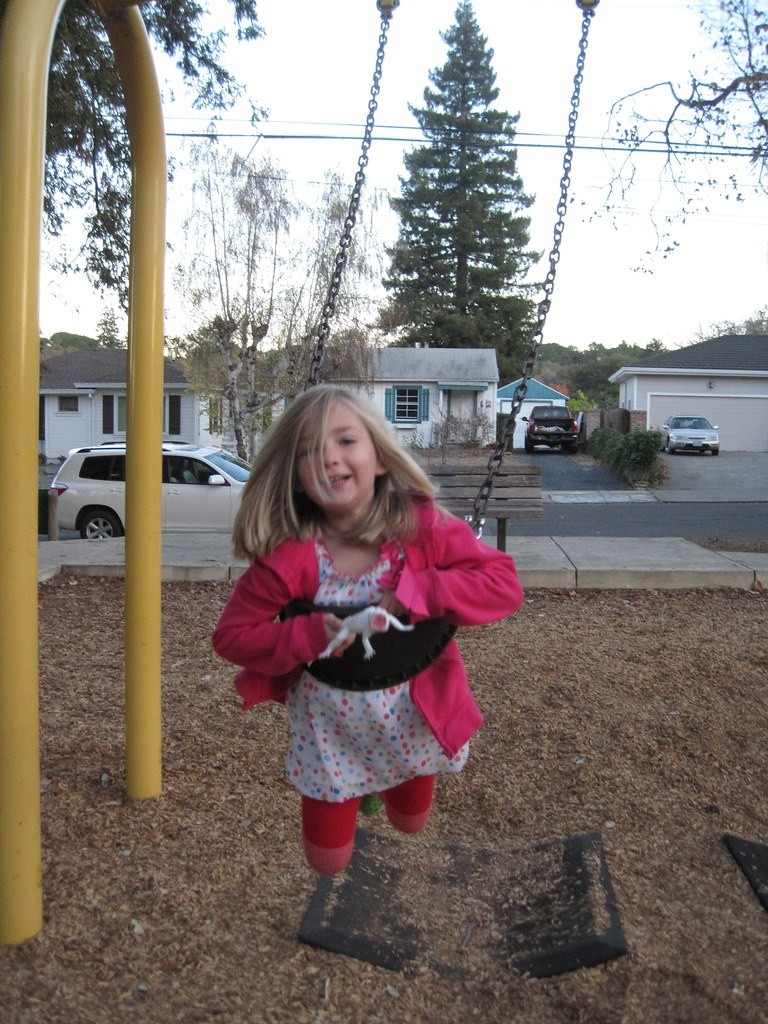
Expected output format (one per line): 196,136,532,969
209,381,527,877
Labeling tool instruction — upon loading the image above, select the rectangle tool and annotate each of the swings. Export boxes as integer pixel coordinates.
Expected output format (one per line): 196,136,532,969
275,0,603,695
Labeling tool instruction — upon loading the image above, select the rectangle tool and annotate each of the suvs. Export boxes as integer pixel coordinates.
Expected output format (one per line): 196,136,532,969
48,438,256,540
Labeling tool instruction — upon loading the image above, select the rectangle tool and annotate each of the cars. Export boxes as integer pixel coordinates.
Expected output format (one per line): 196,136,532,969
659,414,721,457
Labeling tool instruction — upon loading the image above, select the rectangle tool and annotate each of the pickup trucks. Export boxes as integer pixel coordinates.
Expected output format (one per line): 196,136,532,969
522,406,584,454
